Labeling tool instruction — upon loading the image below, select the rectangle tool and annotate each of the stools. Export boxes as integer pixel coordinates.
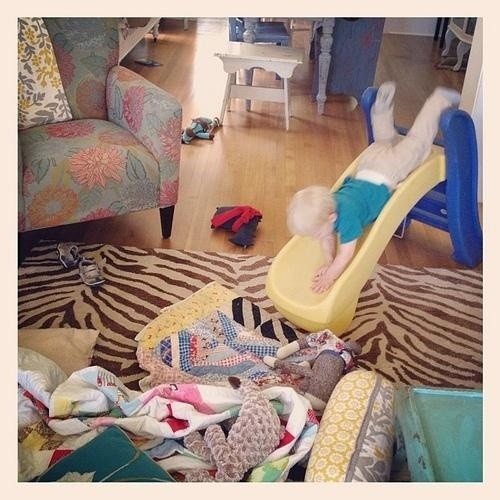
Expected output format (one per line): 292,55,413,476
214,40,307,135
440,17,474,70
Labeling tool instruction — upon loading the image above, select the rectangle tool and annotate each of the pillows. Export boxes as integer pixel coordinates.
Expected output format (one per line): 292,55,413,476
302,368,403,481
17,21,72,129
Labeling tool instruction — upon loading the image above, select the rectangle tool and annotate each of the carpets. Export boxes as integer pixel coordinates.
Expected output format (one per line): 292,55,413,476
19,240,481,395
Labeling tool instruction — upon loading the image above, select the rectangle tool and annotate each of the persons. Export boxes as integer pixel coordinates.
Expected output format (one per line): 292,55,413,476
287,81,461,294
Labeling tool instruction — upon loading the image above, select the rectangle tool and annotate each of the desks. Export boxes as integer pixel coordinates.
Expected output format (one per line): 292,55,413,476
240,17,335,115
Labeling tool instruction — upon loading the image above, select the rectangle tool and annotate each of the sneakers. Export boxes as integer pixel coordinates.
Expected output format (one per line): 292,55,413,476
78,256,106,287
56,241,80,271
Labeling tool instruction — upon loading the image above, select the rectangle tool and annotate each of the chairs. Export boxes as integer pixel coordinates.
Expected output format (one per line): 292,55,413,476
226,18,290,113
18,20,184,247
